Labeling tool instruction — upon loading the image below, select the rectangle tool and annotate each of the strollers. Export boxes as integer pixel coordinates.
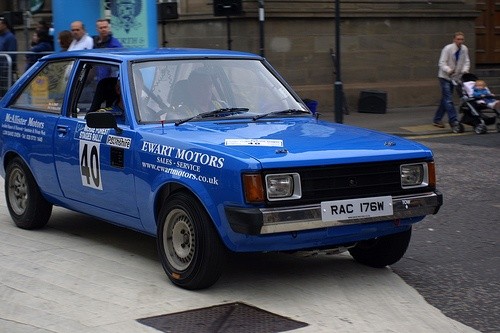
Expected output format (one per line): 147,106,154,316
452,73,500,135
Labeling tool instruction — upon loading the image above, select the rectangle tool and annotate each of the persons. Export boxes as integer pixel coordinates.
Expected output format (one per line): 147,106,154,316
473,80,500,108
433,33,470,127
0,18,18,97
25,19,233,127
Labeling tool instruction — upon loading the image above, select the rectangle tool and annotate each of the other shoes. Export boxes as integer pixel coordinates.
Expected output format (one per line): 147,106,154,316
453,127,463,133
432,121,444,128
494,101,500,109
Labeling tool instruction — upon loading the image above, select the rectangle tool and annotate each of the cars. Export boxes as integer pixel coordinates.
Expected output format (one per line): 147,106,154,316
0,49,444,291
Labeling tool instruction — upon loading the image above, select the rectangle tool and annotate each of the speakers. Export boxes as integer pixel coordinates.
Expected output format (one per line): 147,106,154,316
214,0,242,16
358,91,387,114
158,2,178,20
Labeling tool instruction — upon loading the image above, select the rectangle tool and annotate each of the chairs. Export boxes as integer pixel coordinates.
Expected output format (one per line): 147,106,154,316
86,77,187,114
